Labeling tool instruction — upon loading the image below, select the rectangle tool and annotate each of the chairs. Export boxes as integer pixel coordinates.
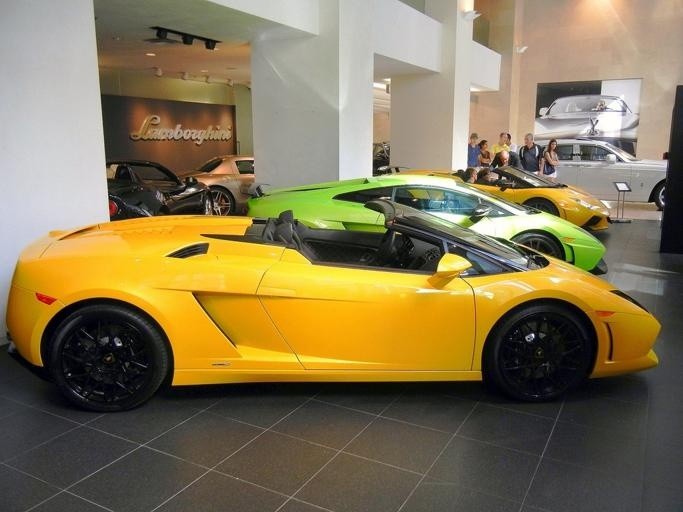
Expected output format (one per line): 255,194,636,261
278,209,320,260
274,223,321,261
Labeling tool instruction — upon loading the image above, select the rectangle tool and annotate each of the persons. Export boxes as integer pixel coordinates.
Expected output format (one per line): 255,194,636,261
477,140,492,169
505,134,520,153
517,133,544,176
461,167,479,184
489,133,511,153
543,139,560,179
477,169,494,186
596,100,607,111
467,133,481,167
489,151,522,170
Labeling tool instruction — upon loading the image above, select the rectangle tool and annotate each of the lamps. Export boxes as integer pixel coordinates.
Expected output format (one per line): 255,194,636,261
153,67,251,90
152,25,221,50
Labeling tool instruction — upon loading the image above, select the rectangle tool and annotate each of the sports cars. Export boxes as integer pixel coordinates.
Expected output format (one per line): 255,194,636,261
105,155,216,217
375,161,611,233
243,173,609,275
174,154,255,216
5,198,661,411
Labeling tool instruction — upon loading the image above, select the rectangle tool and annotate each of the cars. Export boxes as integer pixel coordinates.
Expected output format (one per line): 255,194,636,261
533,93,640,159
373,140,390,169
505,139,668,209
